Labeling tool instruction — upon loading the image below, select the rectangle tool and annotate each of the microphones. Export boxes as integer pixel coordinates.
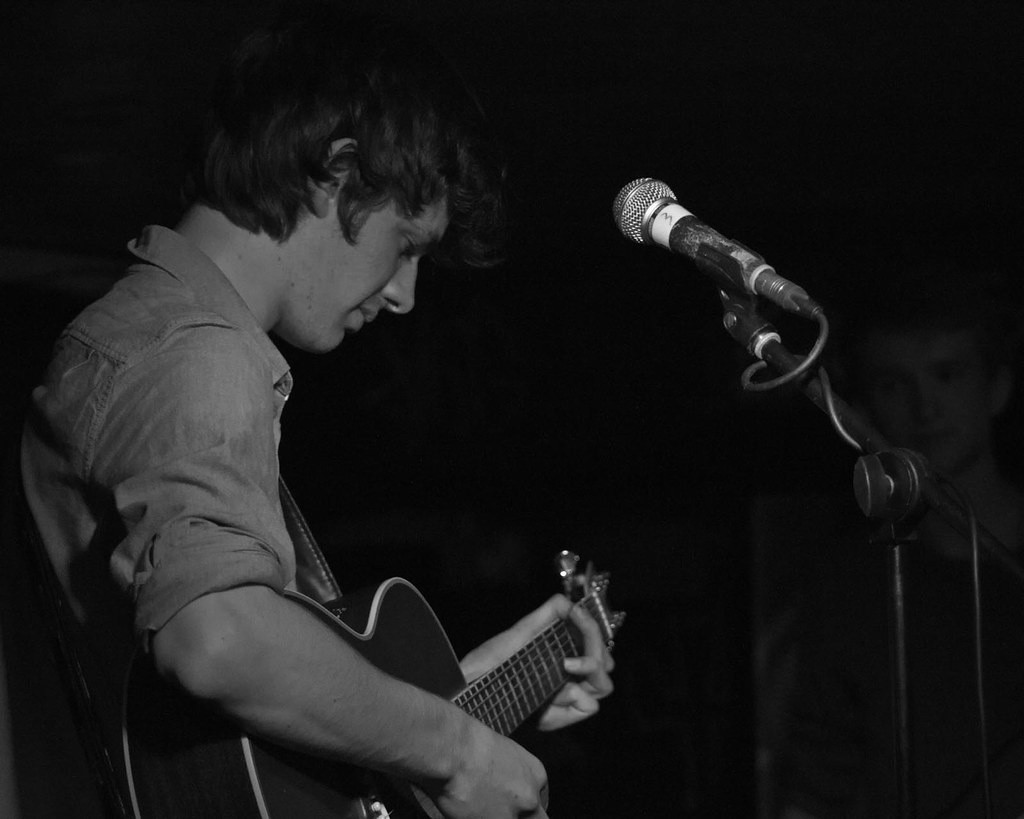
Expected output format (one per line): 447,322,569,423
613,176,824,322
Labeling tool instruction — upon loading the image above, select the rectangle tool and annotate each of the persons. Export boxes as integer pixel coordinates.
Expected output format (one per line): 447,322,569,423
756,274,1023,819
22,35,617,819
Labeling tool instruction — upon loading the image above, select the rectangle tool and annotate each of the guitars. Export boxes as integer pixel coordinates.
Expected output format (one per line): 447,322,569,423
97,547,629,819
